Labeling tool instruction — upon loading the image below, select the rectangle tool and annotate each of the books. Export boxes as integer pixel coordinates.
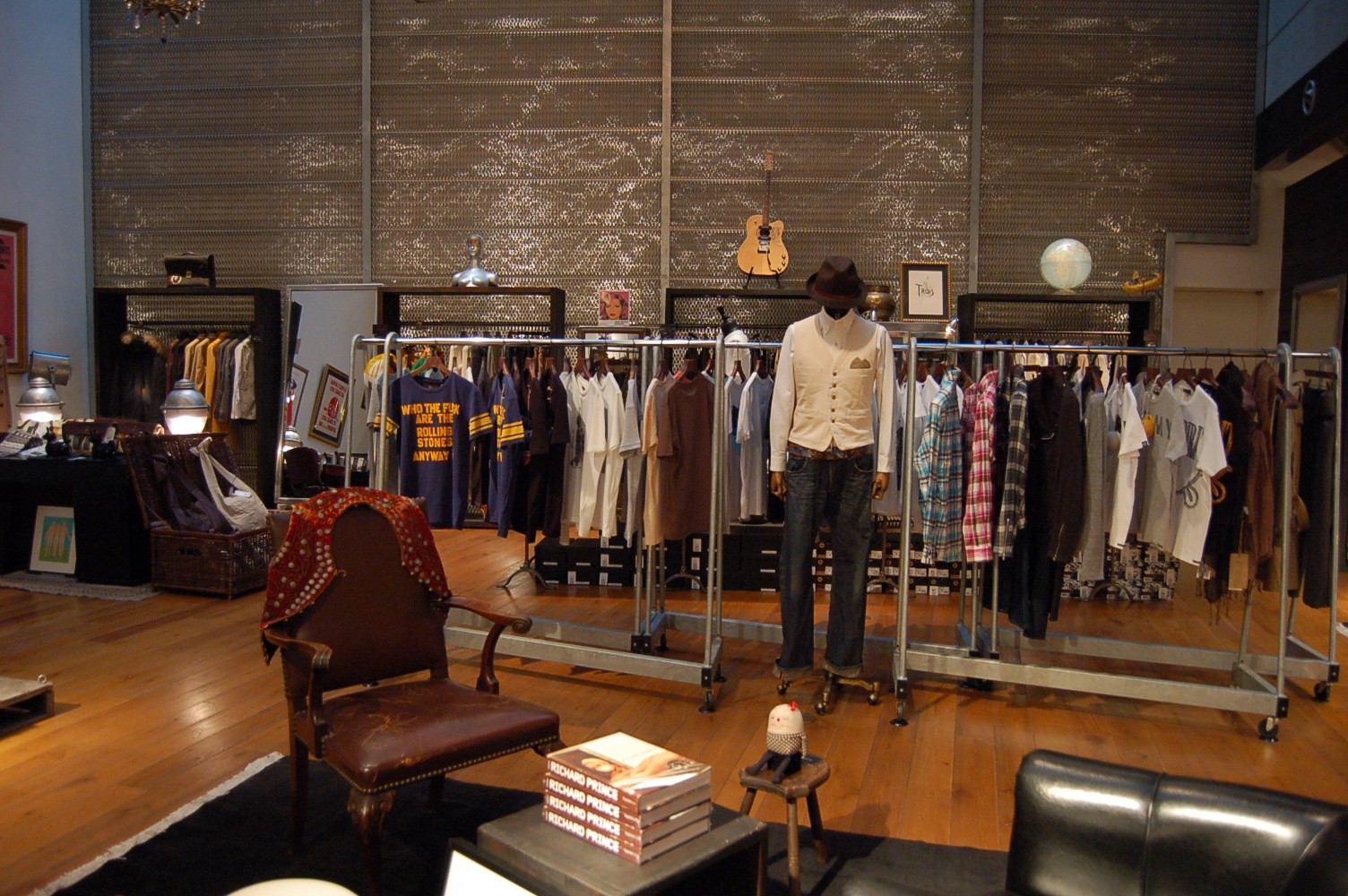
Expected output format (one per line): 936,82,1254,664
542,732,712,865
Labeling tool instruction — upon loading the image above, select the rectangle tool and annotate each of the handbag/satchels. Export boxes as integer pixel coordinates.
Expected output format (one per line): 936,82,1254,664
167,457,229,534
189,437,268,532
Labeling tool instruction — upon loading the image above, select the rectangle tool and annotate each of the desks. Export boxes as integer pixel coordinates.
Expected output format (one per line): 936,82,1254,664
0,431,153,589
475,799,771,896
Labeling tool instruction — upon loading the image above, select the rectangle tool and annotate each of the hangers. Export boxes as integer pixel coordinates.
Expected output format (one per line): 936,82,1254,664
395,322,1339,405
120,317,256,342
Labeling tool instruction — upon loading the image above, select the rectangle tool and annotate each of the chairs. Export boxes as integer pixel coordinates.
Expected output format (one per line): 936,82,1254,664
1004,748,1348,896
261,485,579,896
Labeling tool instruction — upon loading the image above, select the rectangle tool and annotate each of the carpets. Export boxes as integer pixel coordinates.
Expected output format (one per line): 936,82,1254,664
25,748,547,896
0,564,167,601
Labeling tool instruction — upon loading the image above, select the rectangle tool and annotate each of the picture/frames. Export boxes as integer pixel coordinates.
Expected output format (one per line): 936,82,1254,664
0,216,30,375
900,261,952,323
26,502,77,578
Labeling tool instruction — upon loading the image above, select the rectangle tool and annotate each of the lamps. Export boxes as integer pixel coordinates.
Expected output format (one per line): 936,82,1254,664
159,379,213,436
14,376,65,424
26,350,73,410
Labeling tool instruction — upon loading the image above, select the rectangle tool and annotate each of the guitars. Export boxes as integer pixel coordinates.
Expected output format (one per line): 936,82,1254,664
736,149,791,277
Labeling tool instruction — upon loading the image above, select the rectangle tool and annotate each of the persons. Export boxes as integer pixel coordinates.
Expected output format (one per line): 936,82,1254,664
770,255,898,681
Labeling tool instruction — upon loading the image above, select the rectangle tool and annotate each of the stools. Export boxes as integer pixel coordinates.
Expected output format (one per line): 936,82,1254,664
737,750,833,896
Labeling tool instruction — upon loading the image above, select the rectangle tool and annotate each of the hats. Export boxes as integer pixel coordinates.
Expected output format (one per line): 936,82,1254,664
806,255,868,308
